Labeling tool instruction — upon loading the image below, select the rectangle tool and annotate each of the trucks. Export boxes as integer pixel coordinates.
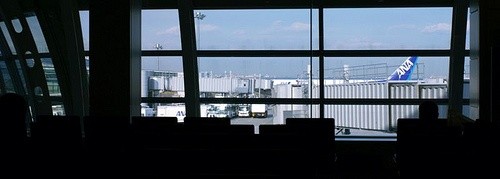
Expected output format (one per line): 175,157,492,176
140,105,155,117
206,104,268,118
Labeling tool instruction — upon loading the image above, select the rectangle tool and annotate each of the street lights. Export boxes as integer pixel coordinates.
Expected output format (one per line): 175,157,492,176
194,12,206,69
153,43,164,71
